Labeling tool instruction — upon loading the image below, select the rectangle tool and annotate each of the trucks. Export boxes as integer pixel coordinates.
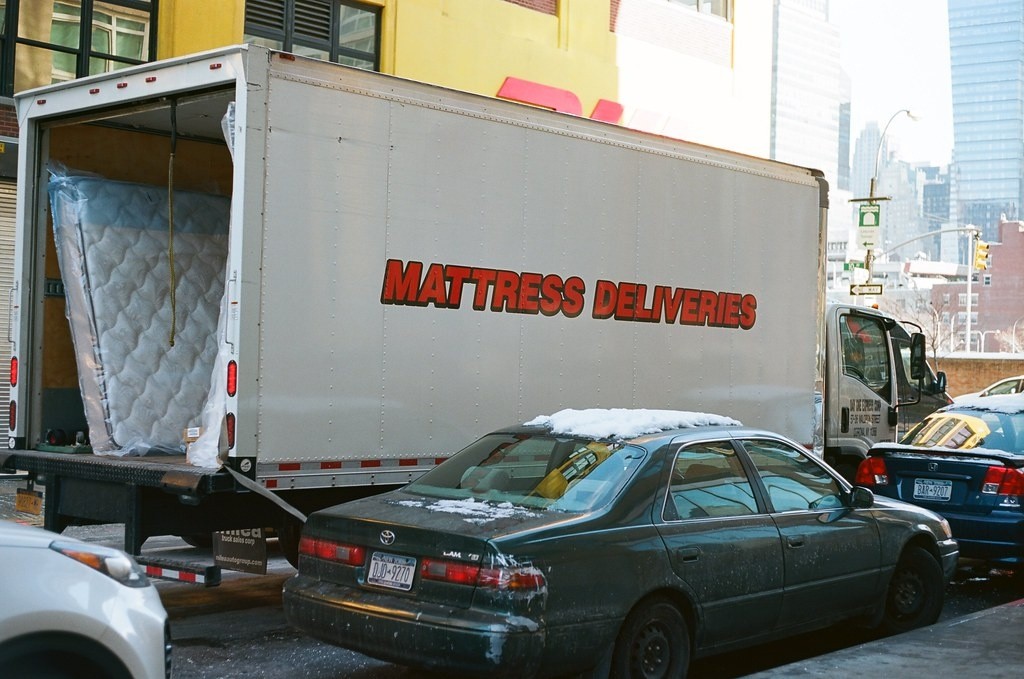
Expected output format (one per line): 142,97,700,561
4,46,966,589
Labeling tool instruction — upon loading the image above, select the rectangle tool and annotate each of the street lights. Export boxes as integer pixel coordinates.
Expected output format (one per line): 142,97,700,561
863,108,916,327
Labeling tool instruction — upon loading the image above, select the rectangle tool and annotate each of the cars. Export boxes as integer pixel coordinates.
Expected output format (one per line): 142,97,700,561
850,393,1024,586
280,405,960,679
949,375,1024,409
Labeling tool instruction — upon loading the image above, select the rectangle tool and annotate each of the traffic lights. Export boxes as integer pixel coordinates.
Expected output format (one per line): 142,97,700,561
978,241,991,269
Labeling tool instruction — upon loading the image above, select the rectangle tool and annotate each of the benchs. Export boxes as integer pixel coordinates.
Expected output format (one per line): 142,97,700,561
463,468,616,507
